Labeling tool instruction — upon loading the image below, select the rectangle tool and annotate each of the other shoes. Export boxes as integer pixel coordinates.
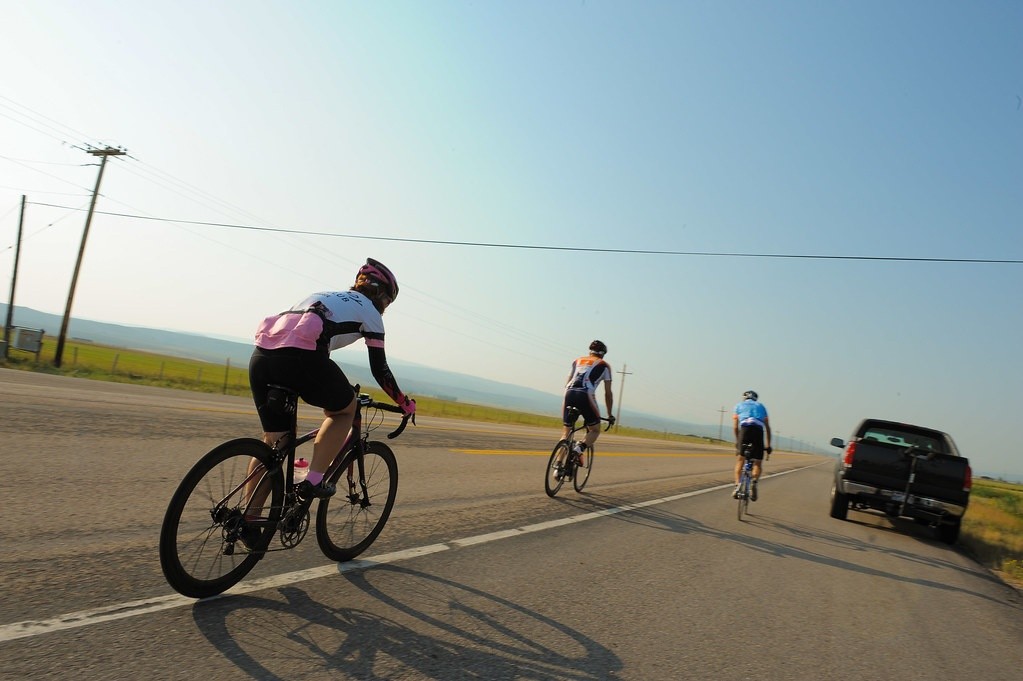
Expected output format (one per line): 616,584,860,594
750,483,758,503
731,483,743,500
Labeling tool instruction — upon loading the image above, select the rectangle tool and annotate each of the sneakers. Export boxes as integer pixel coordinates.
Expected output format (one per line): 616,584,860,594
298,479,336,497
572,446,584,467
553,466,560,482
238,517,262,551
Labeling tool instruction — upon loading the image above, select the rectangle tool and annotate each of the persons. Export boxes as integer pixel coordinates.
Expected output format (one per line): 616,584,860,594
553,340,615,484
732,390,772,501
243,256,415,548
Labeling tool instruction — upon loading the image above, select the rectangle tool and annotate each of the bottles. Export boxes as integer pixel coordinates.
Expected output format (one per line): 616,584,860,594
571,440,576,448
293,458,309,485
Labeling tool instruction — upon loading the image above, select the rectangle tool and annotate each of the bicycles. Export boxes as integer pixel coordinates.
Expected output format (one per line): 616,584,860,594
544,405,615,498
157,382,418,599
733,445,768,522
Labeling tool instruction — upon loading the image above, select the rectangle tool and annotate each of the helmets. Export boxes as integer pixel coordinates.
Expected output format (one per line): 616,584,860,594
741,389,759,401
589,340,607,355
355,257,399,303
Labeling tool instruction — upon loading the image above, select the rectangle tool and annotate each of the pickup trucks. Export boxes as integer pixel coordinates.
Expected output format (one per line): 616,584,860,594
829,418,973,549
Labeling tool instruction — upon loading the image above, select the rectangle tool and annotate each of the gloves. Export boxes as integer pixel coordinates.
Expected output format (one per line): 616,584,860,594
399,396,417,419
608,416,615,422
